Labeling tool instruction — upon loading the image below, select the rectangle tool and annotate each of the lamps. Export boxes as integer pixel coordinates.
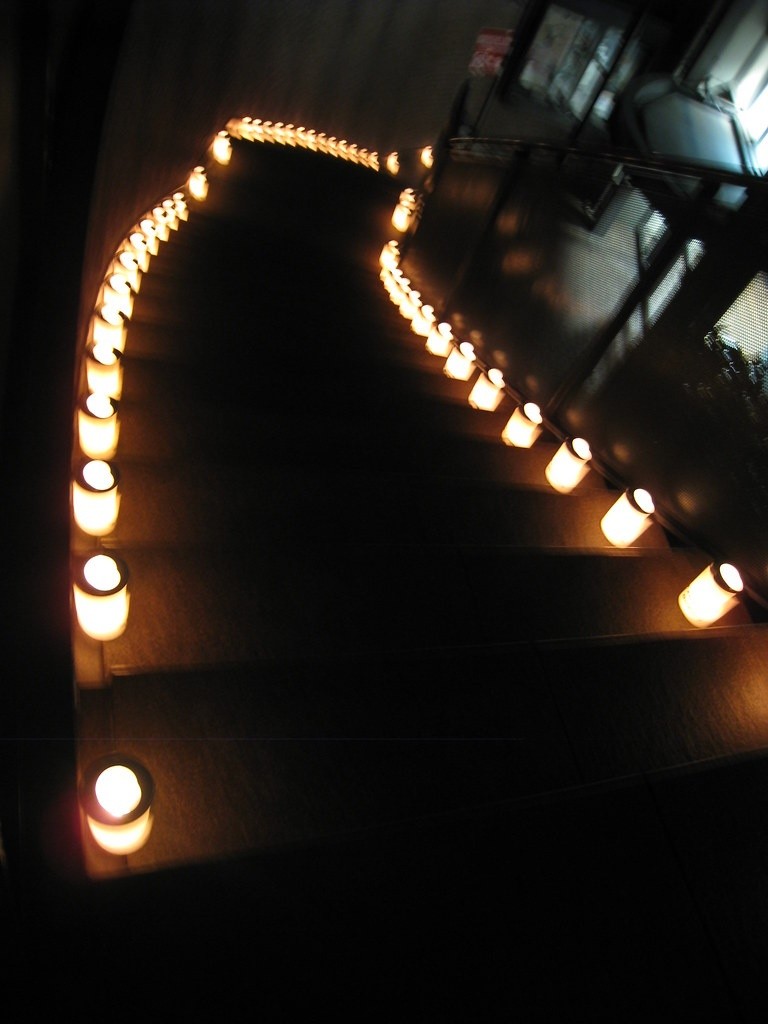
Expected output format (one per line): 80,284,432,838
501,403,546,446
679,563,743,625
72,116,508,460
72,459,123,539
601,489,656,550
71,551,131,643
545,436,594,495
80,751,154,857
